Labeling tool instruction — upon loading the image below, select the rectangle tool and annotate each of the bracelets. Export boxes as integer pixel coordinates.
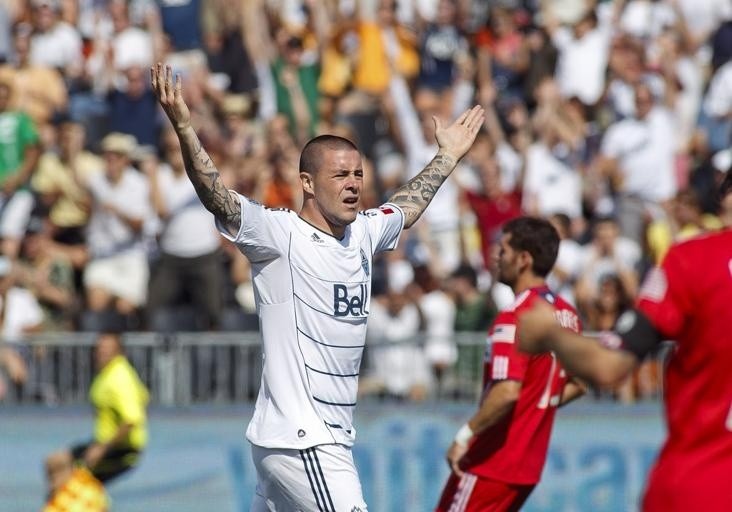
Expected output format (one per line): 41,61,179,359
455,424,475,448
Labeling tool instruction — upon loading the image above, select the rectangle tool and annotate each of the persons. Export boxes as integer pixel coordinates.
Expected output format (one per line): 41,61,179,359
148,61,484,512
43,328,151,494
514,226,731,511
2,1,732,402
436,217,582,511
